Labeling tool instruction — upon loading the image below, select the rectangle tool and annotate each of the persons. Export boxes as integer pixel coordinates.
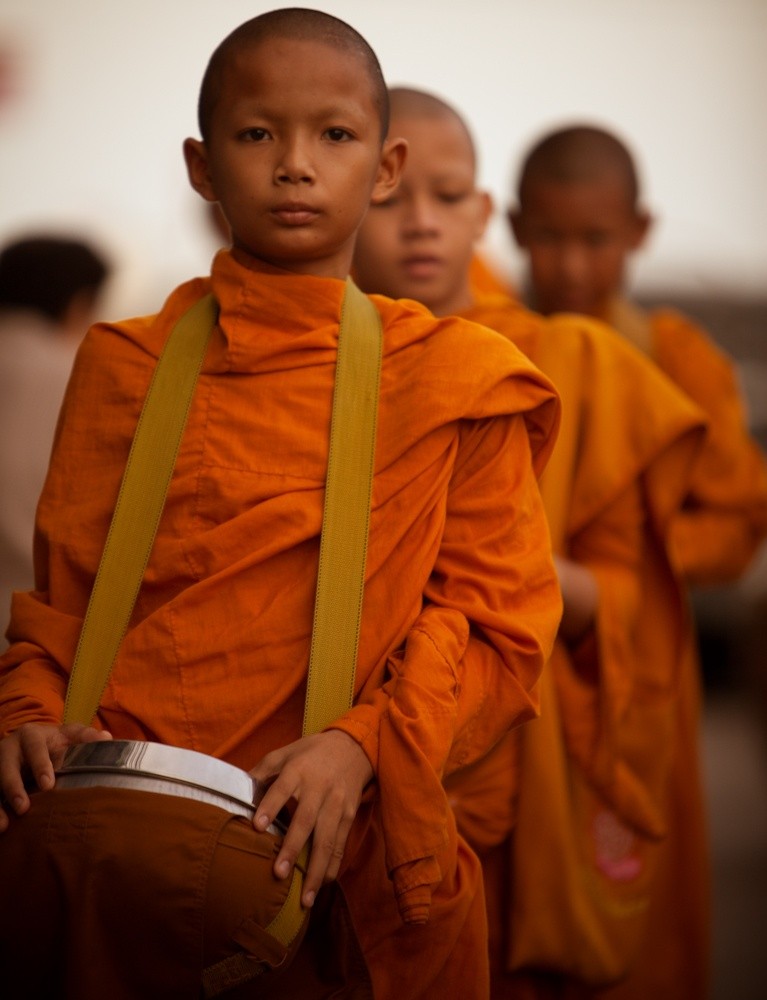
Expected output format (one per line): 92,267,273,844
509,126,766,1000
351,87,712,998
0,233,114,646
1,9,562,1000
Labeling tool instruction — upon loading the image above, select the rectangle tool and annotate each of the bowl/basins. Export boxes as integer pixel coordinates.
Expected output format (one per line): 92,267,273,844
32,738,292,839
